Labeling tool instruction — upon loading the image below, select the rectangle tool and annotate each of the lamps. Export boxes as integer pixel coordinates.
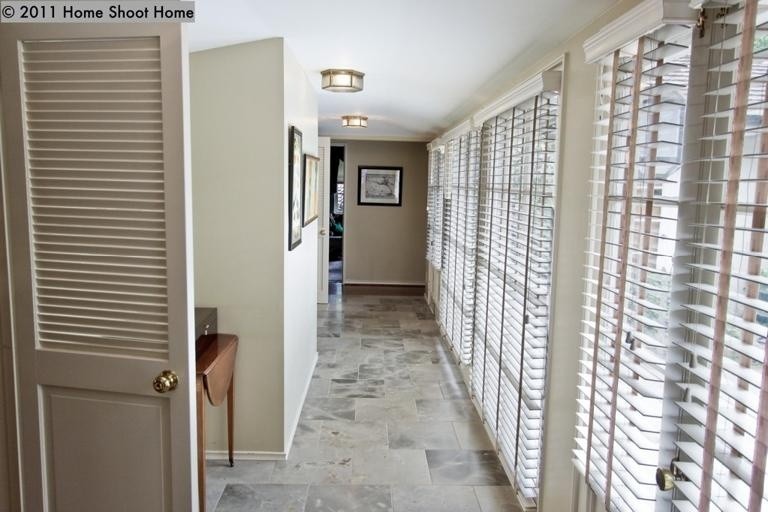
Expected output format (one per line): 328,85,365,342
341,115,368,128
321,68,365,92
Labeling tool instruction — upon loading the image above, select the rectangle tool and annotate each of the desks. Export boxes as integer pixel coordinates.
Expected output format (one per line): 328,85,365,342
196,332,239,511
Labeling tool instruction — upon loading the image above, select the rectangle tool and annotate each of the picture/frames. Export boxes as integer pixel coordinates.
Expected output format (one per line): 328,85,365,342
357,165,404,206
303,154,320,227
289,126,302,250
